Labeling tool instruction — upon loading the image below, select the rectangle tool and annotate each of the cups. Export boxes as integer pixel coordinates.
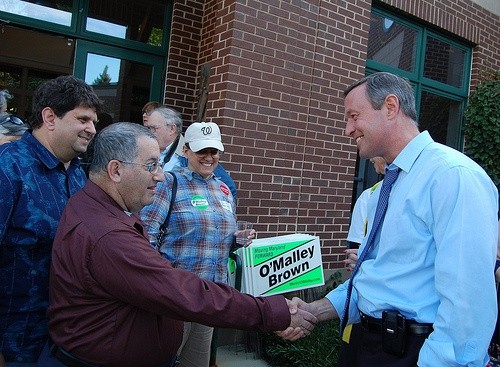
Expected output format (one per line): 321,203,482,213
236,221,253,245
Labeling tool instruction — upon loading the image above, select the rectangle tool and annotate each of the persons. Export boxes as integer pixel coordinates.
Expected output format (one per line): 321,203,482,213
274,72,500,367
0,77,103,367
0,89,238,203
37,122,319,367
138,122,255,367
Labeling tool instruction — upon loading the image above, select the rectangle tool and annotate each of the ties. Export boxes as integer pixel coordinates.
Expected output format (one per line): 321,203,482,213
337,170,401,340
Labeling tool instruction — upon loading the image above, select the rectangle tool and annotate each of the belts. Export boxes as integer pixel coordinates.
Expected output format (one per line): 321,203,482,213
44,338,185,367
354,321,434,337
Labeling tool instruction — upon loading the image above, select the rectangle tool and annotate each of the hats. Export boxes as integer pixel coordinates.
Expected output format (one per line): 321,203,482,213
183,121,224,153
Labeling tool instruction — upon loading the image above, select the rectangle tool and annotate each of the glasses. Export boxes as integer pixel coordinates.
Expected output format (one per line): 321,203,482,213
195,150,223,159
0,115,32,125
142,110,153,117
145,124,172,133
118,160,166,175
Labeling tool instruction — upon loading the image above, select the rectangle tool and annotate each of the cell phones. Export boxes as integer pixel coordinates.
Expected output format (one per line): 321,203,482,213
382,310,406,357
